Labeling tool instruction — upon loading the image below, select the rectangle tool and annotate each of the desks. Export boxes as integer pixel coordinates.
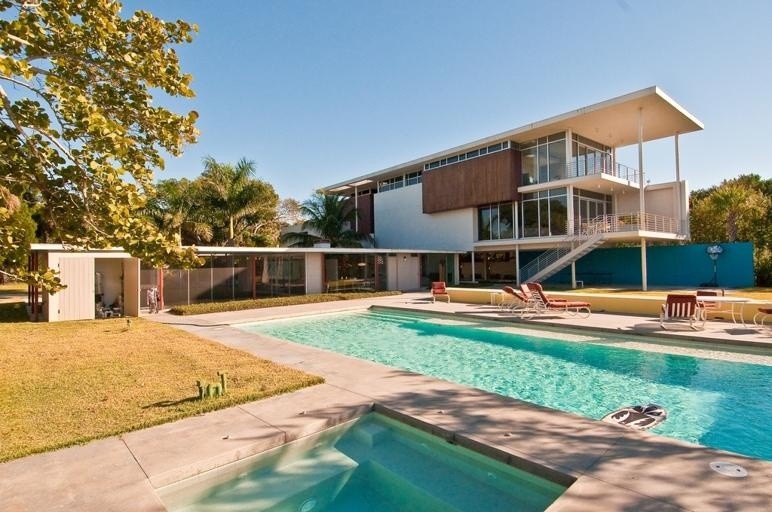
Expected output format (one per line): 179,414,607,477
694,296,752,331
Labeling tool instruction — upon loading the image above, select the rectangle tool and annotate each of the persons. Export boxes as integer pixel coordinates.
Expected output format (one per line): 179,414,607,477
148,287,155,314
155,291,161,313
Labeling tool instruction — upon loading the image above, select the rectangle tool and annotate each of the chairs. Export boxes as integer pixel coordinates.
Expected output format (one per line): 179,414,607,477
658,294,700,330
519,284,567,314
694,290,718,323
501,285,568,317
752,307,771,330
527,283,591,319
431,282,450,304
